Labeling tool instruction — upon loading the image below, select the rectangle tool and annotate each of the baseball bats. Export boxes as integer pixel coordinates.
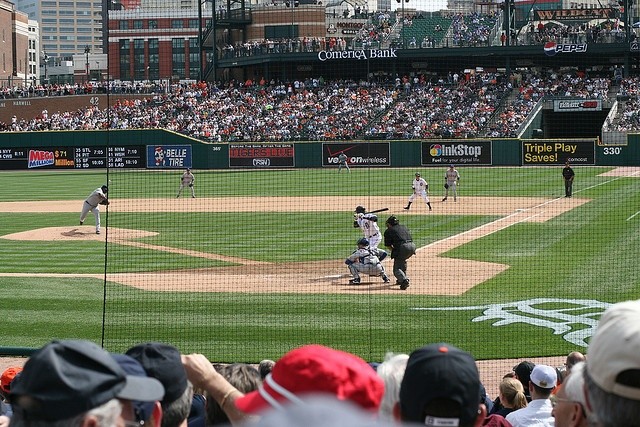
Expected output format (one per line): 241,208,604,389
354,207,389,218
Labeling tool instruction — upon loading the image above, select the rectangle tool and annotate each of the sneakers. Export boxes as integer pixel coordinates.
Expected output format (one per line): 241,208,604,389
442,198,447,201
396,281,401,285
404,207,409,209
177,195,179,197
429,208,432,211
80,221,83,225
565,195,568,197
569,196,571,198
192,195,195,198
400,280,410,289
349,278,360,284
96,230,100,234
454,200,456,202
384,278,390,283
379,252,388,261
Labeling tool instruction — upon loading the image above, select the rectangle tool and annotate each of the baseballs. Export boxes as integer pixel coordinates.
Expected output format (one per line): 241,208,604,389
108,179,111,183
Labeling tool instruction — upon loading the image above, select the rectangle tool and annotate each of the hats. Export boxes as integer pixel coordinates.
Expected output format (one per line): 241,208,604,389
12,338,166,421
126,342,187,408
109,353,155,422
340,151,344,154
451,164,455,167
565,162,569,165
233,344,386,422
399,342,479,427
10,370,24,394
415,172,420,176
587,299,640,400
513,361,535,381
531,364,558,389
0,367,25,393
187,168,190,171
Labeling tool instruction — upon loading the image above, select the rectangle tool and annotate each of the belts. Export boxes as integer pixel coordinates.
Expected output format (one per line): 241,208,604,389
396,240,412,246
369,232,378,238
86,201,92,206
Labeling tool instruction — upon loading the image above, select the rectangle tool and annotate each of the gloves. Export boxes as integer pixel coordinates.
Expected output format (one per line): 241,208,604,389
353,213,359,222
359,213,365,218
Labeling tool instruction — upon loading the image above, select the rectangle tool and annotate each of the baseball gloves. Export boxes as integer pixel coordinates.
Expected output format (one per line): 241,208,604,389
444,183,449,189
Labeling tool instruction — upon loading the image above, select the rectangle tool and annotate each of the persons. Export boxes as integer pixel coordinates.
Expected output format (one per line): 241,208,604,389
468,69,548,138
80,185,110,235
338,151,351,172
316,70,390,137
354,206,387,261
176,80,236,141
392,8,460,50
60,106,117,131
0,108,60,131
501,1,540,47
544,71,610,99
404,173,432,210
177,168,195,197
0,300,639,427
216,36,347,57
384,217,416,289
611,73,639,136
442,164,460,201
0,78,39,98
236,69,316,139
345,238,390,284
118,80,176,131
563,162,575,198
353,7,397,48
38,75,118,97
448,6,505,47
390,67,468,138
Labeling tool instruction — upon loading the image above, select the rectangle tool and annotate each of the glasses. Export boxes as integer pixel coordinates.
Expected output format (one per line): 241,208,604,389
564,363,569,367
549,393,588,419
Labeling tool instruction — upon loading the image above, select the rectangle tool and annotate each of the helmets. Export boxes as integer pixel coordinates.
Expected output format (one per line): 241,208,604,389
357,237,370,246
102,185,109,191
354,206,366,212
385,216,399,228
